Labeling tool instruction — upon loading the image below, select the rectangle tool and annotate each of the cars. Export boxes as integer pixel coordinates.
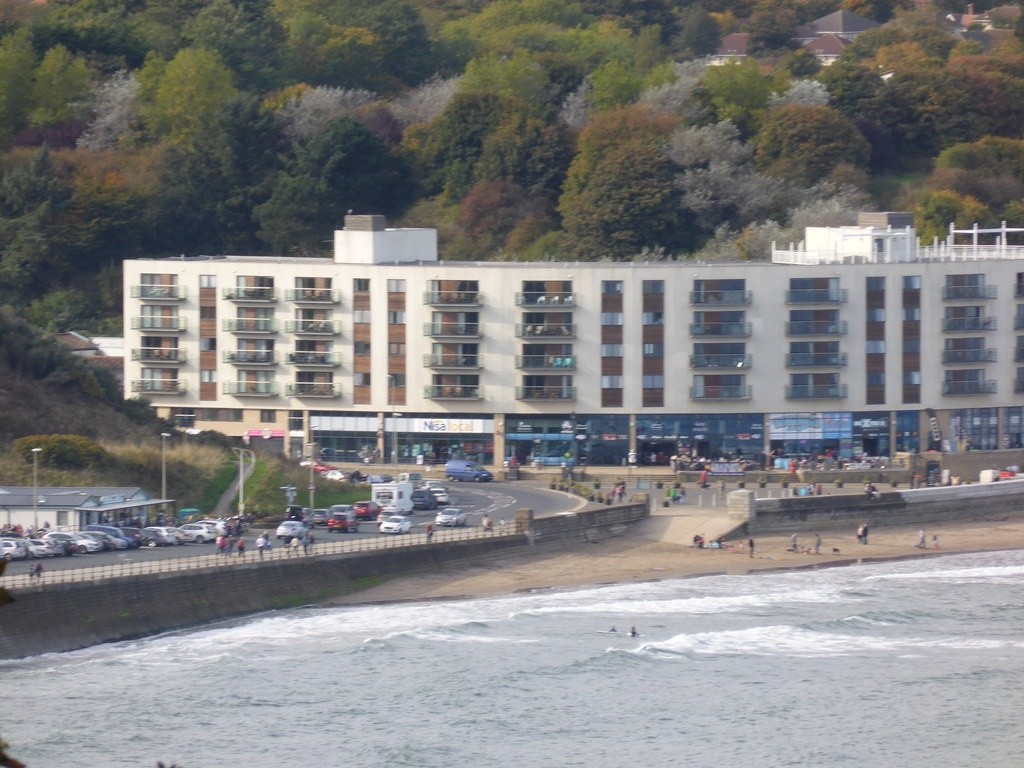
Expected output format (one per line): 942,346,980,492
119,527,161,548
145,526,189,547
434,508,467,528
321,469,353,483
0,526,77,563
297,454,338,475
81,530,127,550
312,508,329,526
368,473,392,485
413,479,449,511
327,513,359,533
83,525,138,550
176,523,217,543
276,521,309,541
42,532,104,554
379,516,412,535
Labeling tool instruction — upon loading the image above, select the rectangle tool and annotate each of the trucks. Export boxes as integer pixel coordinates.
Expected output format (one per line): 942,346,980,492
371,482,414,515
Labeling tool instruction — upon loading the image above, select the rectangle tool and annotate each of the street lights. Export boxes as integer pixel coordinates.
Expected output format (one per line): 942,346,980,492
160,433,171,502
30,448,41,532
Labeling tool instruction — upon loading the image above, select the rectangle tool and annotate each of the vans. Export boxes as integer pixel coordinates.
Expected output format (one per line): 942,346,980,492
389,472,422,487
302,508,314,529
445,460,493,482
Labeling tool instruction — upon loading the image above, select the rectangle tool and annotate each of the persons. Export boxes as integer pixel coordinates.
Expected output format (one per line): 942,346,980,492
858,523,868,544
932,535,937,548
256,535,266,557
749,538,754,558
701,470,707,486
814,534,822,552
482,515,489,530
791,533,798,551
215,534,245,556
865,482,878,498
666,485,686,504
918,528,925,546
289,536,309,554
630,626,639,636
427,523,432,540
800,480,822,496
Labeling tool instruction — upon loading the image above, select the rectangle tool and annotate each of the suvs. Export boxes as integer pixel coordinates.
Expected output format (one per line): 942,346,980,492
197,520,229,538
354,501,380,521
328,504,356,519
